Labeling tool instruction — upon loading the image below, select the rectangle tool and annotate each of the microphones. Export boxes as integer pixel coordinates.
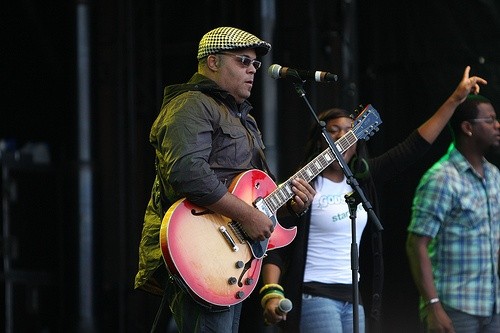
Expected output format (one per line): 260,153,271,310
268,64,337,82
265,299,292,326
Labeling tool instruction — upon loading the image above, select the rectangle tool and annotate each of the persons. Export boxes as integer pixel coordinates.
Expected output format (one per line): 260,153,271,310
134,27,316,333
261,66,489,333
407,96,500,333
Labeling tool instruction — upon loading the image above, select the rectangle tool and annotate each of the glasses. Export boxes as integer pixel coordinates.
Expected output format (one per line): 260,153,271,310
216,51,262,70
469,116,498,125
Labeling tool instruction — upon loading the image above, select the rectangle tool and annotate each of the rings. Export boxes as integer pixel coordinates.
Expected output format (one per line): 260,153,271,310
303,195,309,203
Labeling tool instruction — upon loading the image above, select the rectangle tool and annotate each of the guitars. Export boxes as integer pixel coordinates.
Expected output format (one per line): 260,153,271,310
159,104,383,312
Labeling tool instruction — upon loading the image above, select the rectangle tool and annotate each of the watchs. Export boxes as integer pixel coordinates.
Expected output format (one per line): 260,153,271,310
294,209,313,219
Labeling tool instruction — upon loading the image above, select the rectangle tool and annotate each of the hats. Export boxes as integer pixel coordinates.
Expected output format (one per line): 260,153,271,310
197,27,272,60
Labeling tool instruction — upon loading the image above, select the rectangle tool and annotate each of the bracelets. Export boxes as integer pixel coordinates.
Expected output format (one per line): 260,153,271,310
419,298,443,315
258,284,286,310
